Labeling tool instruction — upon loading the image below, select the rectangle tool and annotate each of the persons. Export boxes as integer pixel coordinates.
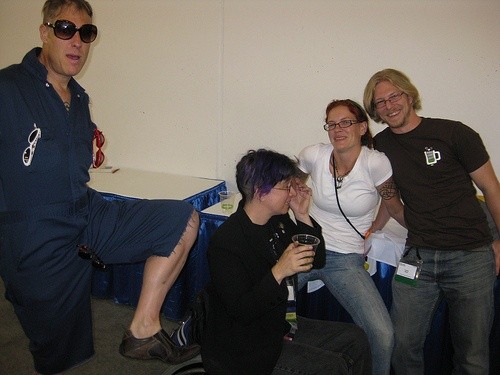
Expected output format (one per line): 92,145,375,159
196,147,327,375
287,99,411,375
362,69,500,374
1,1,201,375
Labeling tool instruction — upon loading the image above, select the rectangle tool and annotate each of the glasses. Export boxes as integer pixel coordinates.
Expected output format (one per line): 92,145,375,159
91,128,105,168
324,120,360,131
22,123,41,166
44,20,97,43
374,91,405,110
77,243,111,272
271,181,295,190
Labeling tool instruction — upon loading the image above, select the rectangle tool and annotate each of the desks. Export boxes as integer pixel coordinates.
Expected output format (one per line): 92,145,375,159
84,165,228,321
201,193,391,280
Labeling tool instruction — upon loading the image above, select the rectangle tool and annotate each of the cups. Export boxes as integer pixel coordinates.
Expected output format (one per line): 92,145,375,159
291,234,320,272
218,191,237,216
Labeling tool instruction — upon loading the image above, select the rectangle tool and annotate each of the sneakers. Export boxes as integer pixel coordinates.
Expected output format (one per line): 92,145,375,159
118,328,202,365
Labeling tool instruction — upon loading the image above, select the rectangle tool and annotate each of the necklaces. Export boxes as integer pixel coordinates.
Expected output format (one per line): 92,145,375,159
333,159,353,183
